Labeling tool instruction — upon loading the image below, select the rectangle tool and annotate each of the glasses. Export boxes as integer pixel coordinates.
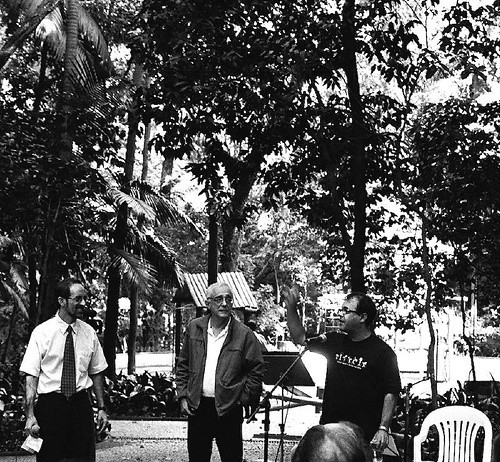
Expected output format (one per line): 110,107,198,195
339,308,363,317
207,295,234,304
65,296,87,302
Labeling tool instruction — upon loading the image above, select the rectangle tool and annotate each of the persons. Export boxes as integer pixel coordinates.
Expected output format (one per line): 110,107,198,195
18,278,108,462
290,423,366,462
247,322,267,351
175,282,265,462
284,288,402,462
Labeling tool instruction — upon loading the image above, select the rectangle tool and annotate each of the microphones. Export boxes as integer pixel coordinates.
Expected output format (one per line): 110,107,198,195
306,334,327,343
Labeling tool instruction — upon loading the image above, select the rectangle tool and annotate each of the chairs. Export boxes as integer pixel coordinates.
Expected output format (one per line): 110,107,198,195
414,405,493,462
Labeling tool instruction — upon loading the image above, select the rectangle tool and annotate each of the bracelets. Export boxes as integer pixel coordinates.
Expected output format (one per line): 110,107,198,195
98,407,106,412
379,426,386,430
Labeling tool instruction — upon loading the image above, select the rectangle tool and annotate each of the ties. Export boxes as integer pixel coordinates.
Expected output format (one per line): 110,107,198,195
61,326,76,400
